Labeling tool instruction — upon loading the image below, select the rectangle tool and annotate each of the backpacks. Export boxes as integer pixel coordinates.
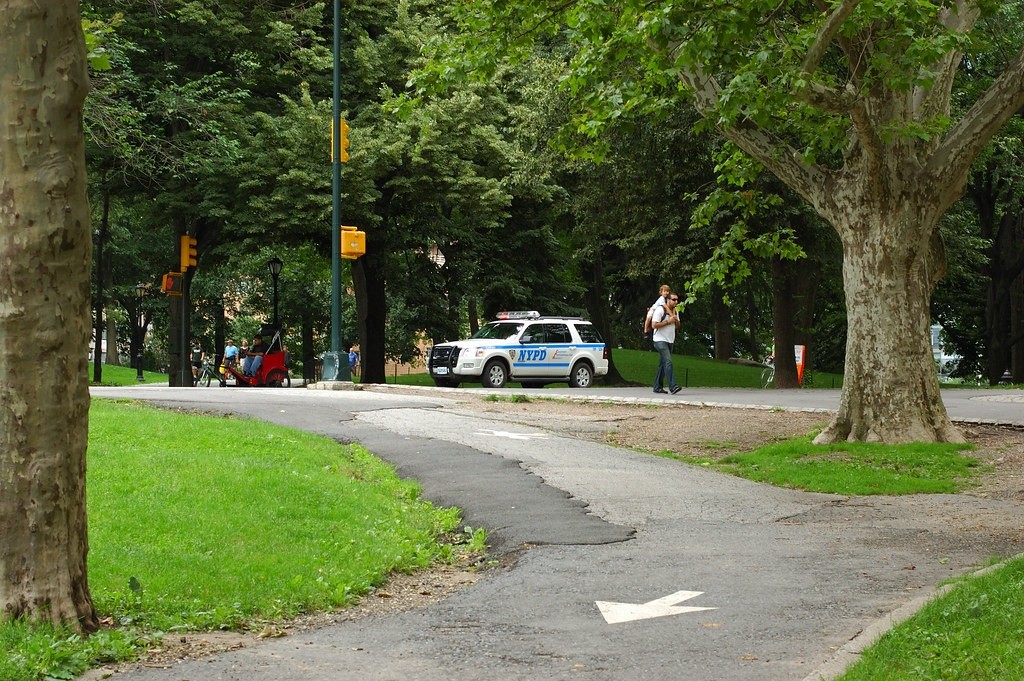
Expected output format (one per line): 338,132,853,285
649,305,666,342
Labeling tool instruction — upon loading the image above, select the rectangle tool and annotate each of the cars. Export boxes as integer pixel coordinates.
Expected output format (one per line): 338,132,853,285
930,324,1014,380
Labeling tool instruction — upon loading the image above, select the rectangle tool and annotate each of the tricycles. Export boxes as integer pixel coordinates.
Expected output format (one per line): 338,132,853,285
194,323,292,388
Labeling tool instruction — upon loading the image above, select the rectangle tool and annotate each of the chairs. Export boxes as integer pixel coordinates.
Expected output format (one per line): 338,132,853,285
255,351,284,376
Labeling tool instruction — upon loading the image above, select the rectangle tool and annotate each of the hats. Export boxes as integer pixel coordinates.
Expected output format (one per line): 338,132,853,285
225,340,233,343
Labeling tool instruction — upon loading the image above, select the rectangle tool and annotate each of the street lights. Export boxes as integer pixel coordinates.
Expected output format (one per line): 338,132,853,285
134,283,147,382
268,255,283,327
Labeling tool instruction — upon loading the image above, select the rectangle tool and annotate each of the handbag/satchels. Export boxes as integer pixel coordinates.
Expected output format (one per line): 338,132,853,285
219,363,225,374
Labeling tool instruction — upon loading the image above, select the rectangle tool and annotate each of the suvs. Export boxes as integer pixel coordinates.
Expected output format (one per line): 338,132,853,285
426,310,609,389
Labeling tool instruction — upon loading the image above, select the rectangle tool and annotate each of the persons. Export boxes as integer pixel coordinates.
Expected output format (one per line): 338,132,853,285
190,342,205,385
348,348,357,378
643,285,676,339
241,334,273,378
222,340,239,380
651,292,682,395
282,345,292,387
238,339,250,375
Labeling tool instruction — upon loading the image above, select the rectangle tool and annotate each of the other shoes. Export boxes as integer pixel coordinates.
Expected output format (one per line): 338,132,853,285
643,333,648,338
671,386,682,394
653,388,668,394
242,373,253,378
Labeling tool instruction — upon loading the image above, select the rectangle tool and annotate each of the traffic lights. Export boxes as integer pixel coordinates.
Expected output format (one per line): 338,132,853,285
332,115,351,166
180,235,198,269
162,271,183,296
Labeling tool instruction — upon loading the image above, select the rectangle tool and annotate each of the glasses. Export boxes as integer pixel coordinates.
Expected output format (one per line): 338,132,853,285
668,298,678,301
